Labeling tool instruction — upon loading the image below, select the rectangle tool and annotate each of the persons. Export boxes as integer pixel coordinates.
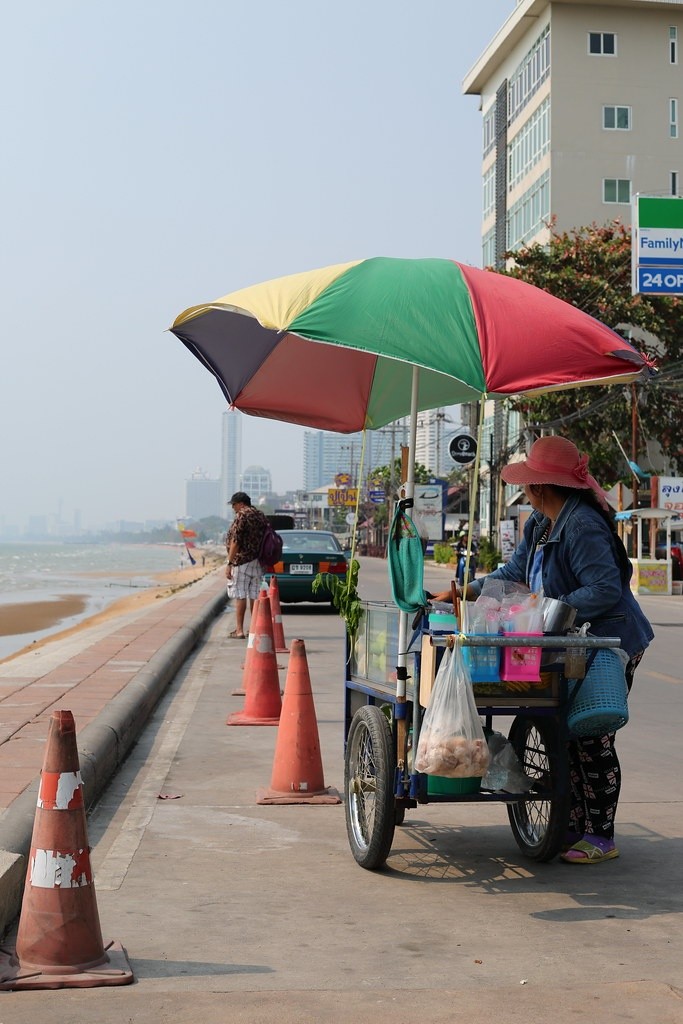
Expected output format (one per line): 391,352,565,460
426,437,655,863
225,491,267,639
454,531,476,582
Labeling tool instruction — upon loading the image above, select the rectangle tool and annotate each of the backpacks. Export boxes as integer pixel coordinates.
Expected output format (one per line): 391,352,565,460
251,508,283,565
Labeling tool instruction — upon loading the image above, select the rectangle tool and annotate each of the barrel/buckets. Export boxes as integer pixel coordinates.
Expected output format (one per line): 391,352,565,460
542,597,577,634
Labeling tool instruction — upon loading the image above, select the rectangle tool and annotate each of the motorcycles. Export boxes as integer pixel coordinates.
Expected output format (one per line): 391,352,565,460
456,549,476,585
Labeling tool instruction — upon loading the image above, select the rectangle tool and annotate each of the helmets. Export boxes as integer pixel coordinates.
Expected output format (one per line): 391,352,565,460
458,531,466,538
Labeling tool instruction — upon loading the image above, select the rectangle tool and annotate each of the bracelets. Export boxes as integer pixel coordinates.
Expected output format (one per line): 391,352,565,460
458,588,462,596
227,562,233,565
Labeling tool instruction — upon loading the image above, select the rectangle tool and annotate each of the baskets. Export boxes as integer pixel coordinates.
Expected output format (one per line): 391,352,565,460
565,639,629,736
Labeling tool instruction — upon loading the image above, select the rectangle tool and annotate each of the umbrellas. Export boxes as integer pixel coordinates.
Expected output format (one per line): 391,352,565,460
166,256,648,697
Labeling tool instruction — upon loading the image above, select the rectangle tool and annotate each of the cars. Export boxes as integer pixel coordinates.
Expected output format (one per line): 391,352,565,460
265,529,350,611
640,542,683,579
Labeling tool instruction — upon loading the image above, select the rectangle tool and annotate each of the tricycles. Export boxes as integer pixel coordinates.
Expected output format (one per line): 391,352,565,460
341,598,621,868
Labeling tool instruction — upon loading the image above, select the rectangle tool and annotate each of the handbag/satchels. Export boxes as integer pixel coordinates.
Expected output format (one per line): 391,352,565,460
415,636,490,776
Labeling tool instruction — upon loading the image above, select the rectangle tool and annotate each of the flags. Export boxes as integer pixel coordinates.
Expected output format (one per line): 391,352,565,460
186,542,195,549
178,523,196,538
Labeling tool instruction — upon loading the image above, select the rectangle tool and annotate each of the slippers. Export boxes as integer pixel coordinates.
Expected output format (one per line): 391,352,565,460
229,629,245,639
560,840,619,865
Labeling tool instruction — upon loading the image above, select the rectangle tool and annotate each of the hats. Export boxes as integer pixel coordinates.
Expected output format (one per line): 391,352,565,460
227,492,251,504
500,435,615,513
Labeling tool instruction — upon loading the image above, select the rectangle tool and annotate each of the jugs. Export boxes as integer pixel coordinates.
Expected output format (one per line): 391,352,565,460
429,610,456,633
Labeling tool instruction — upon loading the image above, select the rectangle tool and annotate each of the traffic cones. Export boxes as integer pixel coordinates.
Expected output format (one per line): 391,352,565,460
229,596,281,727
0,708,135,987
255,640,342,804
241,575,292,670
